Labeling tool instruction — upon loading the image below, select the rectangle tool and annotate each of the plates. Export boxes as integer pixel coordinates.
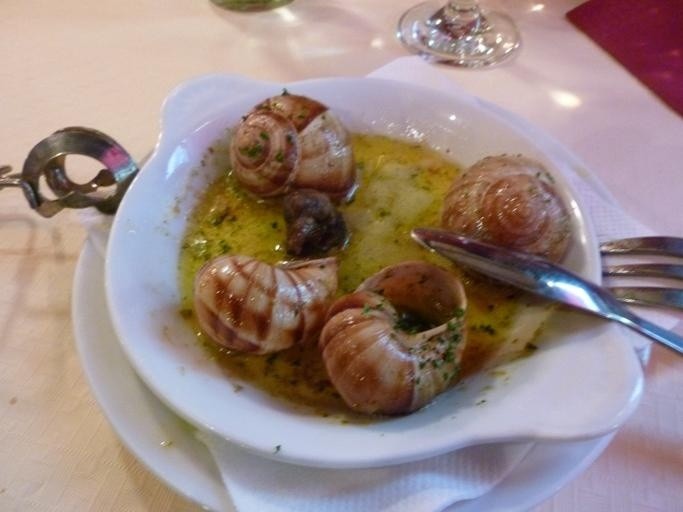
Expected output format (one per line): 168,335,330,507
67,88,652,511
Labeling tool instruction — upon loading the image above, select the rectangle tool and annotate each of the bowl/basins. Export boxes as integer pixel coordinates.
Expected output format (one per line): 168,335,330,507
101,69,647,473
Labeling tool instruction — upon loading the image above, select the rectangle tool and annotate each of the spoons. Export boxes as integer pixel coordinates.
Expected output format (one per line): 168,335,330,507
408,227,682,358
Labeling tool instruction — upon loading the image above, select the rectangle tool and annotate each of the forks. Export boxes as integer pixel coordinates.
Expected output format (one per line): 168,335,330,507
598,235,682,308
